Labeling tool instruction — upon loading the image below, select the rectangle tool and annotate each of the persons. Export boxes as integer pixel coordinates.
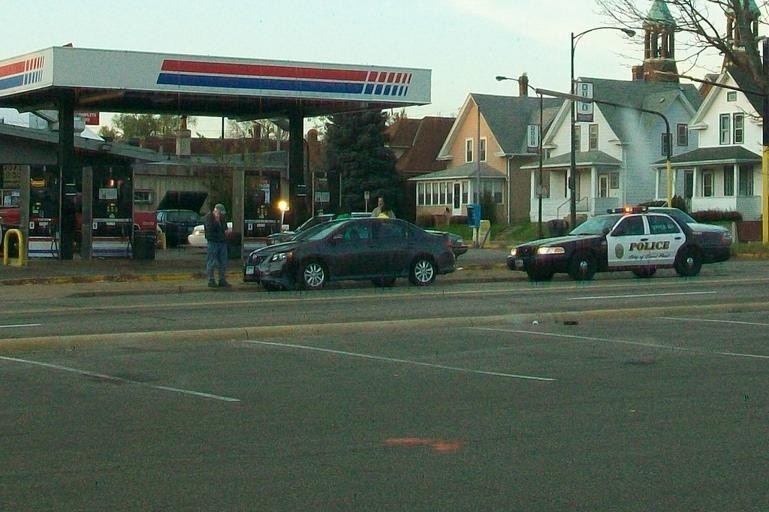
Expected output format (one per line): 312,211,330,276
203,202,233,289
371,197,395,220
376,203,391,219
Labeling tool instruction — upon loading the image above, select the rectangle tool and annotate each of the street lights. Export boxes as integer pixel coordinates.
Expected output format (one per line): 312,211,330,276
646,71,769,243
495,76,543,234
569,27,635,230
537,88,673,208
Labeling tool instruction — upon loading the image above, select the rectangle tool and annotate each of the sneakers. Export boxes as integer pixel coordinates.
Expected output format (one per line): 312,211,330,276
208,279,232,288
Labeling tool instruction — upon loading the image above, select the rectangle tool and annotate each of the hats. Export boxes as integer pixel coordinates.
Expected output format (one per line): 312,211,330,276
215,203,226,214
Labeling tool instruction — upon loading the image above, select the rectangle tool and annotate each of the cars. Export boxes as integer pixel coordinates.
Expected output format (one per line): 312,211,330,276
506,205,731,280
242,213,468,290
155,210,201,246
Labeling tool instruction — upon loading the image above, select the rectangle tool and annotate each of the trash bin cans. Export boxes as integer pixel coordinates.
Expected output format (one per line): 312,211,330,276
134,230,155,260
547,219,569,238
